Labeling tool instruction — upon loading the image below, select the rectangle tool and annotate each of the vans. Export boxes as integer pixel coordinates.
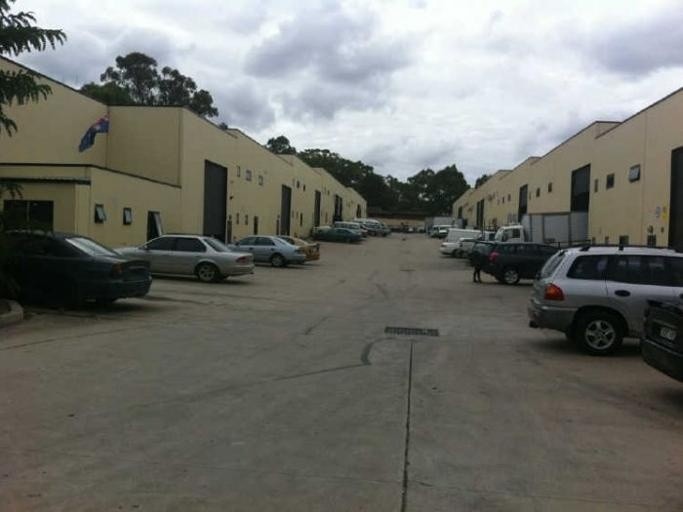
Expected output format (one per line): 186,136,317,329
528,243,682,356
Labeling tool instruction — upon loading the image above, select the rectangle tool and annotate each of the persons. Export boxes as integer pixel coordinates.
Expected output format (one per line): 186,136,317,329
469,246,486,283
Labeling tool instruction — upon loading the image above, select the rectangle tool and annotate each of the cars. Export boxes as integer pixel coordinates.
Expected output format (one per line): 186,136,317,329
0,229,152,308
113,234,254,283
425,211,589,283
228,235,320,267
313,218,391,243
639,292,682,383
